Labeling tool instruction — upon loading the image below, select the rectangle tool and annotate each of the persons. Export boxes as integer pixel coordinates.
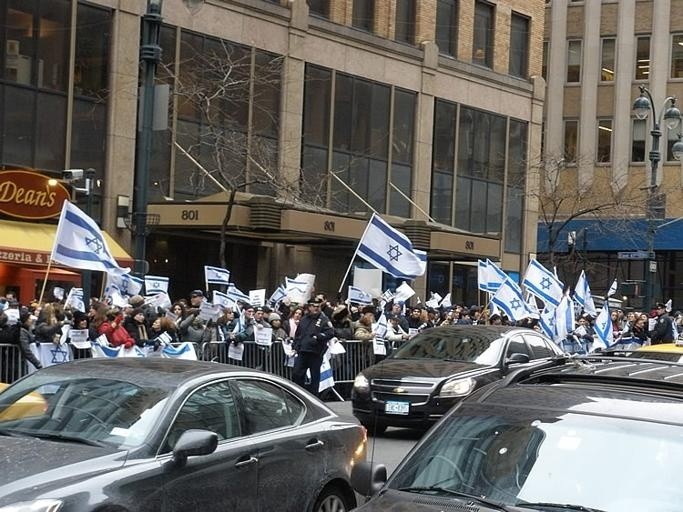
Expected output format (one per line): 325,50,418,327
1,294,683,392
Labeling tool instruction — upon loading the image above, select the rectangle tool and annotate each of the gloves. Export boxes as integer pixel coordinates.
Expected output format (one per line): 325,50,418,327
231,332,249,346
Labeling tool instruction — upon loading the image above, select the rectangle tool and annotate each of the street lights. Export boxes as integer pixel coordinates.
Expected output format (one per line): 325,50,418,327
632,83,683,313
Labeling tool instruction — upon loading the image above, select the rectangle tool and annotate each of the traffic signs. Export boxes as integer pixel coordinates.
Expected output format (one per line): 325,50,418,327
617,252,657,273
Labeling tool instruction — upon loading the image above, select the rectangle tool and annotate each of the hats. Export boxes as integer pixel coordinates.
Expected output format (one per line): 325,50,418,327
411,304,422,315
307,298,323,307
129,294,145,311
655,303,667,309
188,289,203,297
241,303,253,312
426,307,438,314
131,308,144,318
268,312,282,323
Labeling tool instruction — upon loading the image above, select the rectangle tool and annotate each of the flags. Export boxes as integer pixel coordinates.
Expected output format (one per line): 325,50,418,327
357,214,426,280
51,200,132,275
481,258,616,347
51,270,168,313
205,266,454,313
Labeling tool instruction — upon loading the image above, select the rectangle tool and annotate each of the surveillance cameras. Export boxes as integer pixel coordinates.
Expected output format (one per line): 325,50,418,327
61,169,83,181
